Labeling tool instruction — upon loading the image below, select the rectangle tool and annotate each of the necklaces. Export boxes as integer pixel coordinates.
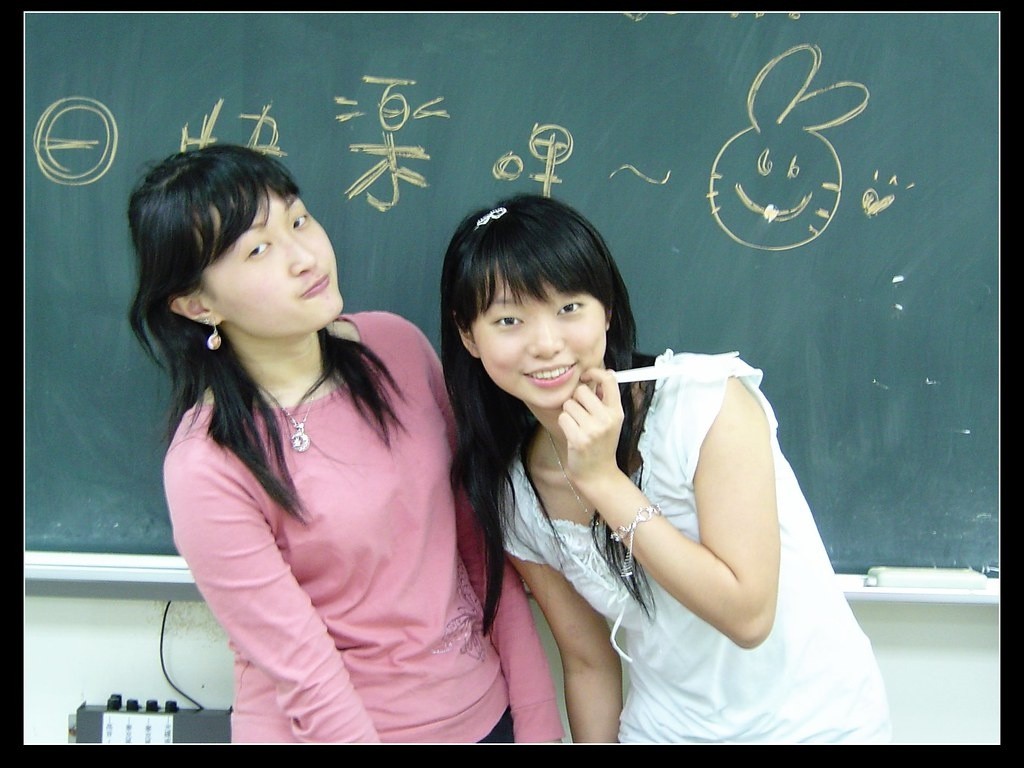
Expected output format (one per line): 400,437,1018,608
244,369,325,453
546,431,604,540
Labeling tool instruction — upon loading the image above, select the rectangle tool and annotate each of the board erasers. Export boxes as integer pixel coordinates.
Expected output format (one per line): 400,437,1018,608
863,567,989,590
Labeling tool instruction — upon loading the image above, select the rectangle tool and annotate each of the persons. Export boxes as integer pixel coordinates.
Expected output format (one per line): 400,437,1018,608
441,195,893,743
126,144,566,742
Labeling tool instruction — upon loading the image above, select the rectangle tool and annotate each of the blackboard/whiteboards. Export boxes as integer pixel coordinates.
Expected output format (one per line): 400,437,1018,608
24,12,999,604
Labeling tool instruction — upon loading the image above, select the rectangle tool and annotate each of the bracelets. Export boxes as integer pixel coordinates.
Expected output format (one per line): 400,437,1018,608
612,506,664,578
